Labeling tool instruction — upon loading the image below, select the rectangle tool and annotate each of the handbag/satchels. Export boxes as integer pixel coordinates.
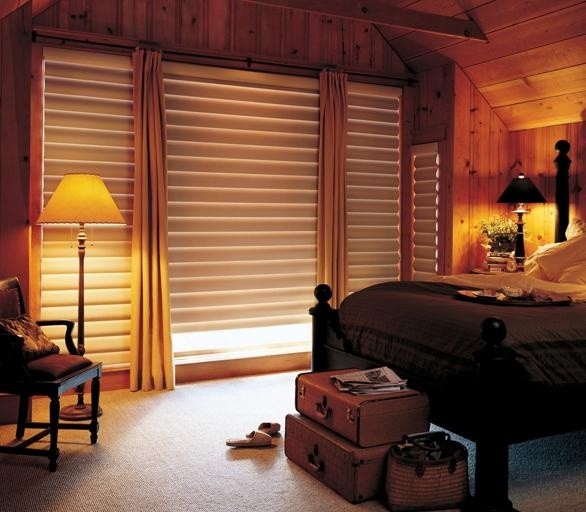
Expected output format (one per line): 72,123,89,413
386,431,471,512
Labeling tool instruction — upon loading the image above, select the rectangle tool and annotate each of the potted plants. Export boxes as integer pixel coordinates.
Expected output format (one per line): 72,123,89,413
475,214,518,271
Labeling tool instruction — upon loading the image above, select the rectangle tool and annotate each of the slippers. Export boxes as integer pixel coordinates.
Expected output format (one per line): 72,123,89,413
225,431,272,446
246,422,281,438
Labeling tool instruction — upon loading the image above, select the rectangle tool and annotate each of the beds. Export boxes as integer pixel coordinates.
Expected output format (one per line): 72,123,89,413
308,139,586,512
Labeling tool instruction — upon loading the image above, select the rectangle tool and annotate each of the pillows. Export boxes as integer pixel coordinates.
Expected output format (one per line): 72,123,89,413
0,313,61,363
524,241,586,286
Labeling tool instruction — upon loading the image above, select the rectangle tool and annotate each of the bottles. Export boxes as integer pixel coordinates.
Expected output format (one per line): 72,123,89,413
506,261,516,272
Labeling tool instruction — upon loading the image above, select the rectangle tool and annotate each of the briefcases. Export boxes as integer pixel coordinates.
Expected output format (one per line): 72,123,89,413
284,413,399,505
295,367,432,447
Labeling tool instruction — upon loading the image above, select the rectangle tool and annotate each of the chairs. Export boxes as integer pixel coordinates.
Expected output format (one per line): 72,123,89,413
0,277,103,472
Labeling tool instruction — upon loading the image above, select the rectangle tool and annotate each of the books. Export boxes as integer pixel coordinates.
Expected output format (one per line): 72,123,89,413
330,365,410,397
485,251,515,272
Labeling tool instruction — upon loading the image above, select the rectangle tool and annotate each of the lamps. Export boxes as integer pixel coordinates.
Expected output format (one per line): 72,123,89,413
34,173,126,421
497,173,549,270
